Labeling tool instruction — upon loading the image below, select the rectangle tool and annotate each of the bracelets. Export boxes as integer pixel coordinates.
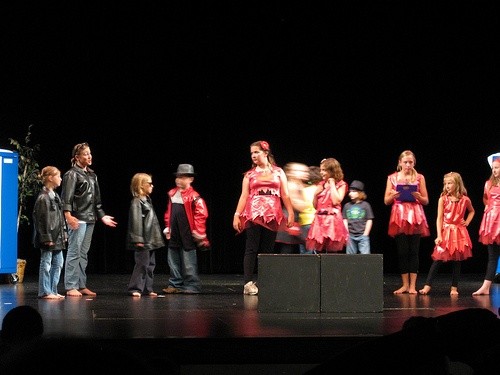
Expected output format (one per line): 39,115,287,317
234,213,240,215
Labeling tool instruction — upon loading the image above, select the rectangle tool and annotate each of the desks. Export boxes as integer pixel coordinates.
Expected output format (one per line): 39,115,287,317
257,253,384,313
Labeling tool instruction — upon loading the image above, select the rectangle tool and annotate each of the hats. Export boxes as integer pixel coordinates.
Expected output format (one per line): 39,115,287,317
347,180,363,191
176,164,195,176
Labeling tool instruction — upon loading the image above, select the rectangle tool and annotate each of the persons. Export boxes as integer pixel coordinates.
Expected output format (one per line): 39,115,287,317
419,172,475,295
384,150,430,294
129,172,165,297
233,141,295,295
33,166,69,299
472,152,500,295
163,164,209,293
275,157,373,254
61,143,118,296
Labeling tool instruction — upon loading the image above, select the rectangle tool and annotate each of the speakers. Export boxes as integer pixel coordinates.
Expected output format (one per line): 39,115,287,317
257,253,385,315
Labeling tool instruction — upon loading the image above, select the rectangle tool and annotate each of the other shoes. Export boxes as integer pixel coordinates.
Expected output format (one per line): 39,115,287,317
162,286,196,294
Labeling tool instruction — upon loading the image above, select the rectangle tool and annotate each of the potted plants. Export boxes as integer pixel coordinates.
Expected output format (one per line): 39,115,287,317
10,123,43,283
